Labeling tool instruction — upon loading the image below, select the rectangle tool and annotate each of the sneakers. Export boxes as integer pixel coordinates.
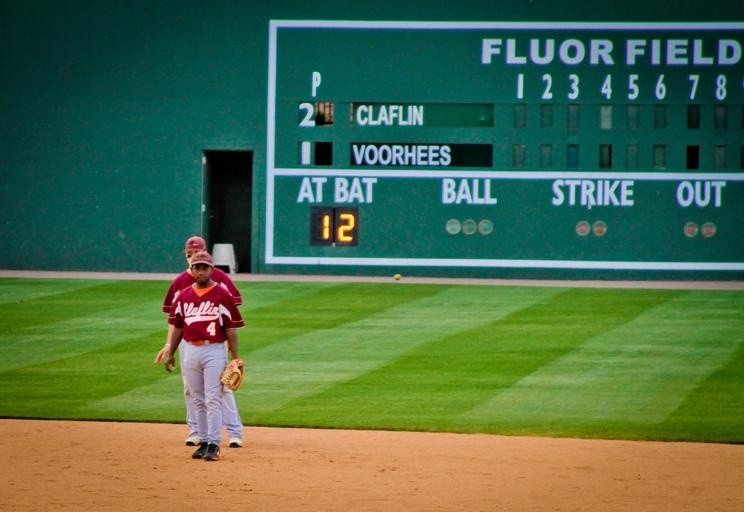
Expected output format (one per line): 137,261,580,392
192,442,207,459
228,437,243,448
185,436,202,445
203,444,220,461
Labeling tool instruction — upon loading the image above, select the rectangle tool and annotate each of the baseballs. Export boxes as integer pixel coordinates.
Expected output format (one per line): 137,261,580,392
394,274,400,279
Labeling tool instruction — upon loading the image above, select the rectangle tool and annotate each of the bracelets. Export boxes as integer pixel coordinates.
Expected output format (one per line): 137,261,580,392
166,343,175,352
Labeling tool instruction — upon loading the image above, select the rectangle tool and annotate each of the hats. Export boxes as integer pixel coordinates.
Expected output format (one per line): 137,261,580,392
189,251,213,267
184,236,207,254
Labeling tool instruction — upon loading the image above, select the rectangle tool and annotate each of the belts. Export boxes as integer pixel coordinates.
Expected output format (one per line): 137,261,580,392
191,339,212,346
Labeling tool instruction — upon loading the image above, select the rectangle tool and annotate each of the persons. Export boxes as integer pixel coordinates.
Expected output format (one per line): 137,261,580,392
153,235,245,447
162,252,245,462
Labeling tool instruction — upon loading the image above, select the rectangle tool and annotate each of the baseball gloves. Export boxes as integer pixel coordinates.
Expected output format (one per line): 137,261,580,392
221,358,245,390
